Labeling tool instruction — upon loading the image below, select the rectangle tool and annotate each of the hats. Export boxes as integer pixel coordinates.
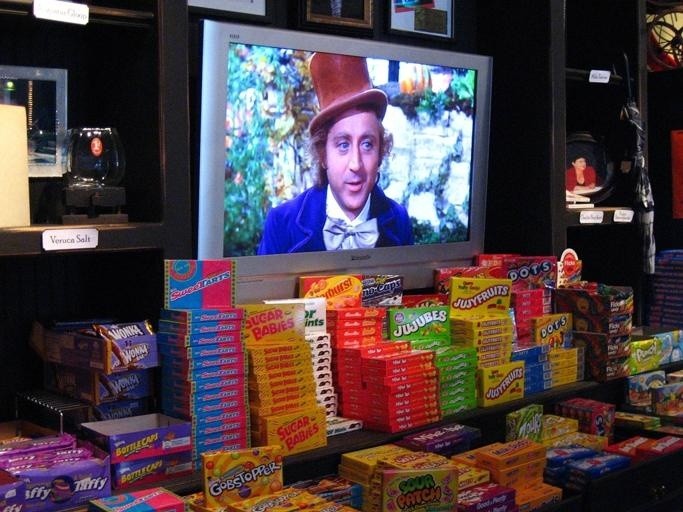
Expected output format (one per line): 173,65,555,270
308,52,389,157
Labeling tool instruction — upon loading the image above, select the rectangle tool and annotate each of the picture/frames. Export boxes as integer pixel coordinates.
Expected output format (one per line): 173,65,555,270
306,0,374,30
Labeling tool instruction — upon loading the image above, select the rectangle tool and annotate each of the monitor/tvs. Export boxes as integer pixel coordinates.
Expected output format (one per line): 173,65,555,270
197,18,493,304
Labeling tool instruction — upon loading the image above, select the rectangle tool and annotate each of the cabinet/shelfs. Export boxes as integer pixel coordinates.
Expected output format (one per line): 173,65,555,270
0,0,682,512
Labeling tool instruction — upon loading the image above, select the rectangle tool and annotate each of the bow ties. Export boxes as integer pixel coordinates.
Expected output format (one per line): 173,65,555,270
322,219,381,251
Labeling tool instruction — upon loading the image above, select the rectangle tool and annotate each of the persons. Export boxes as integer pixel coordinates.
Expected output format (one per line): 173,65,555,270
255,52,415,254
565,154,597,193
